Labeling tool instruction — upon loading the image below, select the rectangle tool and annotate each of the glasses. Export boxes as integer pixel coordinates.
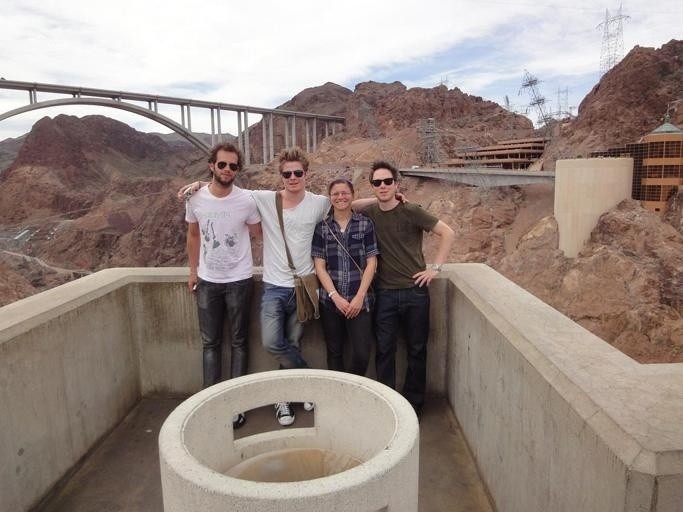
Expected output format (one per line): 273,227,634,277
372,177,394,187
283,170,303,179
217,161,237,170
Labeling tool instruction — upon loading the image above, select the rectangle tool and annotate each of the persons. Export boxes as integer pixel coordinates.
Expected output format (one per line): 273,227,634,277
177,146,410,426
186,141,265,429
356,158,455,420
310,178,380,377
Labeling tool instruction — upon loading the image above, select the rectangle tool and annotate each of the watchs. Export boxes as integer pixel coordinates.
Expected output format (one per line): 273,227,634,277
431,263,442,272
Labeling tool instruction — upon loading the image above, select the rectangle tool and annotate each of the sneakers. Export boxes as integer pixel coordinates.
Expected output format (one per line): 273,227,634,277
304,403,314,412
234,412,246,430
273,401,296,425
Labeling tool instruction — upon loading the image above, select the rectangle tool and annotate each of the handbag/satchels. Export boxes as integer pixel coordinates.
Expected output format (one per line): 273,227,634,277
294,272,321,323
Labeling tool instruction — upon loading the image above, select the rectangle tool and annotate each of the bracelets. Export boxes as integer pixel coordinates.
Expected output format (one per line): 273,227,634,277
198,180,202,189
190,271,198,275
328,290,337,297
